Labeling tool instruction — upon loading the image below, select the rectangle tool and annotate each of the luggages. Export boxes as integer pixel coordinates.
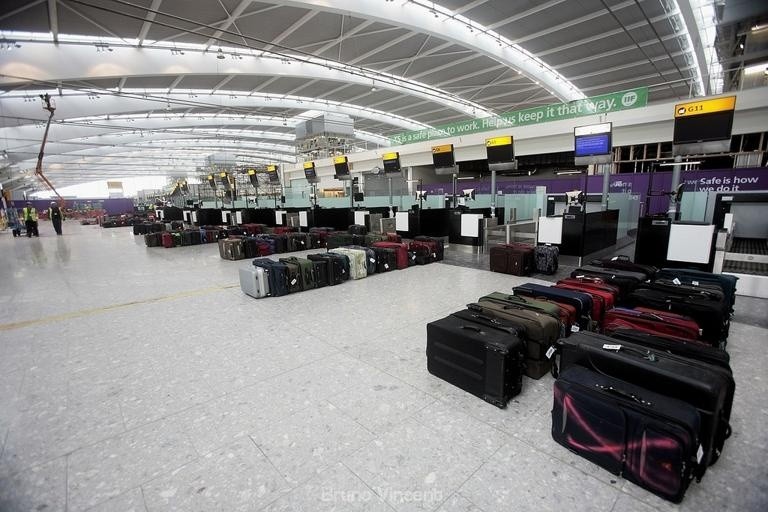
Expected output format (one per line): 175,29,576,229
326,238,443,281
133,219,218,247
425,282,590,411
489,241,561,277
550,254,741,504
217,225,401,261
240,253,330,300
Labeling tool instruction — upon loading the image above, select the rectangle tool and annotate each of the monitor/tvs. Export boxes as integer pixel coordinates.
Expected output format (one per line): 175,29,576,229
673,95,736,145
382,152,401,173
485,136,514,164
333,156,349,176
431,144,454,168
248,170,258,184
303,162,316,178
267,166,279,181
574,122,612,157
208,175,215,187
220,172,229,185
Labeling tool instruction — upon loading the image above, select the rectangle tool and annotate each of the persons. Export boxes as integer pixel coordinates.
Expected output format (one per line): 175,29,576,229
47,201,63,235
103,207,109,222
7,202,39,239
